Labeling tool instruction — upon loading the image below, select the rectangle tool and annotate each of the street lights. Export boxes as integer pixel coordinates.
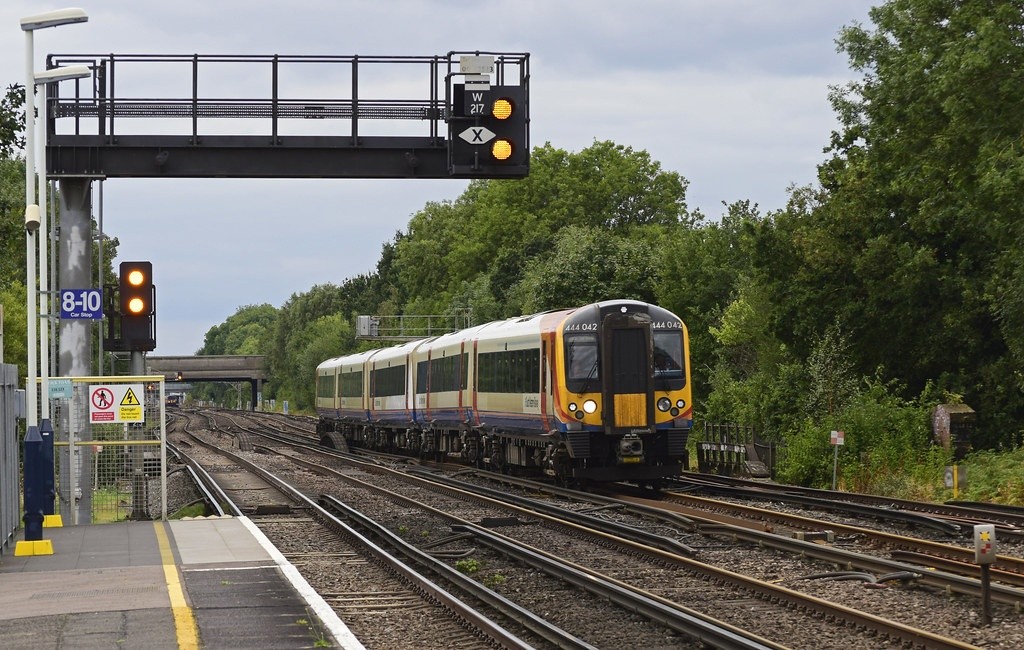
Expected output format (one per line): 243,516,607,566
20,7,90,541
29,63,92,529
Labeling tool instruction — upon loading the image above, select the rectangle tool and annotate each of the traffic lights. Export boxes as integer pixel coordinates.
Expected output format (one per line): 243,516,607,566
119,261,153,316
475,85,530,177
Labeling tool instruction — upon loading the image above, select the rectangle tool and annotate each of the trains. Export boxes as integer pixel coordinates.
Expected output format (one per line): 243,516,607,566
168,396,176,407
315,299,692,490
